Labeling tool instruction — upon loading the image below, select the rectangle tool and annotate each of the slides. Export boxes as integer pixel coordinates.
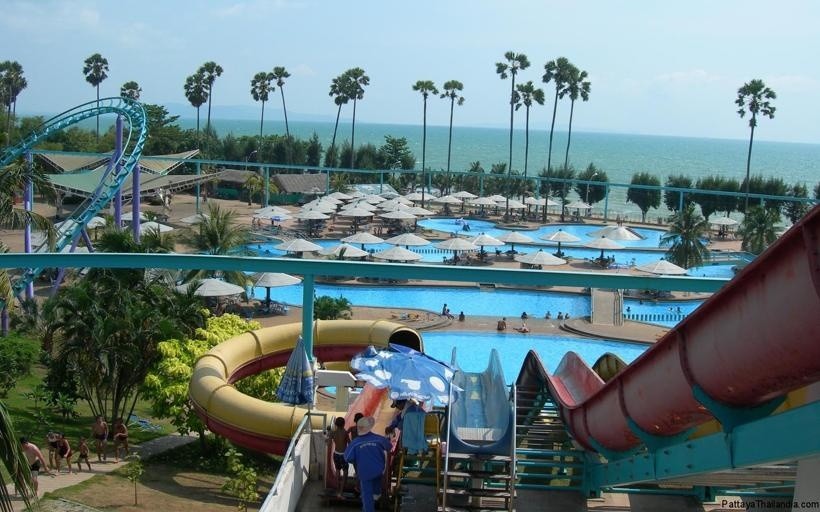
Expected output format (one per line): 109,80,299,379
510,202,818,451
449,346,513,451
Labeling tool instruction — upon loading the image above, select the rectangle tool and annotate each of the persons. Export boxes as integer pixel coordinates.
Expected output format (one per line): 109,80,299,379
346,413,363,493
442,303,572,333
343,416,393,512
385,399,426,504
332,417,351,501
20,415,128,498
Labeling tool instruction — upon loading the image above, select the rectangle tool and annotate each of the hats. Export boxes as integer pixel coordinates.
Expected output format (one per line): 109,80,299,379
356,418,373,436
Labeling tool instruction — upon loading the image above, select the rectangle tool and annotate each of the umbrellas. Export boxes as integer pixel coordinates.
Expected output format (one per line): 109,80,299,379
351,343,465,408
710,217,738,240
275,334,313,405
